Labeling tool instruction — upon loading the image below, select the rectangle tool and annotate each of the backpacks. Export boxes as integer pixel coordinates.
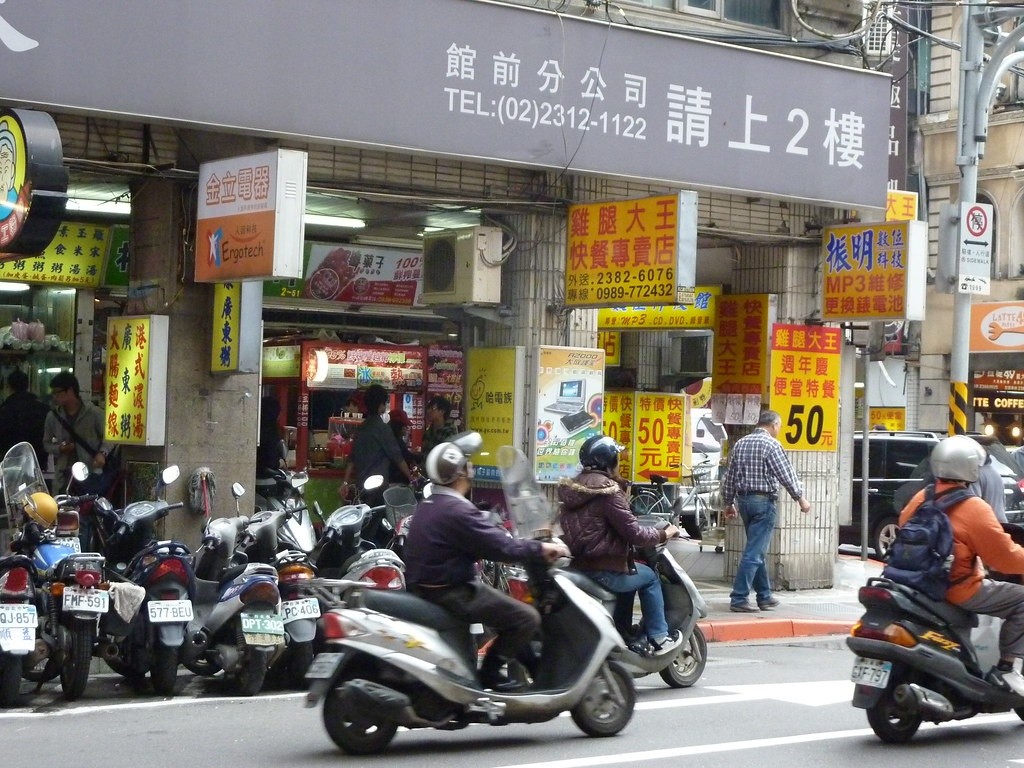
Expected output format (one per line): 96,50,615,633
884,484,979,601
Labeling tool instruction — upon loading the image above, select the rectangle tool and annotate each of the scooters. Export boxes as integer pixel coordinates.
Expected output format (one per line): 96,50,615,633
0,551,39,707
845,525,1024,743
93,439,419,707
499,515,709,687
297,445,637,755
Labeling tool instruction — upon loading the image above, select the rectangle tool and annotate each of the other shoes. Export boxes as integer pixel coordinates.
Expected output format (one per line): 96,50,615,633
479,654,527,693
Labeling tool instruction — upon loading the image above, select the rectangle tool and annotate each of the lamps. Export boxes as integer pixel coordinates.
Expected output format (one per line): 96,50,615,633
982,414,997,435
1008,415,1022,437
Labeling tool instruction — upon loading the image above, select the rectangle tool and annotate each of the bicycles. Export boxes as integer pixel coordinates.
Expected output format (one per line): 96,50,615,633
628,458,711,540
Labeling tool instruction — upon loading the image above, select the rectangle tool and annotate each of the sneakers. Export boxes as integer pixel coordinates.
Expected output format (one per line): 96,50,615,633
757,598,780,609
647,629,683,656
984,666,1024,696
730,602,760,612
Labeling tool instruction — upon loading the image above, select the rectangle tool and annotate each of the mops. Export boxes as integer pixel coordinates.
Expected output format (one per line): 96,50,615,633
185,466,217,518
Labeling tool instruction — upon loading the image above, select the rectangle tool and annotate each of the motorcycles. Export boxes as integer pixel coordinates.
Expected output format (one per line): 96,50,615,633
1,441,120,699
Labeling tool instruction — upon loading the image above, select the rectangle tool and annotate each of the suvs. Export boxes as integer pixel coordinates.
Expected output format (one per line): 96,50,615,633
837,429,1024,564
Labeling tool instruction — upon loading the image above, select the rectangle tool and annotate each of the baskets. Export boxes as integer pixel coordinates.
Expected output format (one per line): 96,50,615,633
683,470,711,494
383,486,418,526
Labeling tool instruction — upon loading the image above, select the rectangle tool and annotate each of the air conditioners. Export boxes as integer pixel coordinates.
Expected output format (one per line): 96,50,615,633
415,225,502,312
859,1,902,64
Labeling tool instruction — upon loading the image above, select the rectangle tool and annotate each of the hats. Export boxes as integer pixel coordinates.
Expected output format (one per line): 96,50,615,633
390,408,414,427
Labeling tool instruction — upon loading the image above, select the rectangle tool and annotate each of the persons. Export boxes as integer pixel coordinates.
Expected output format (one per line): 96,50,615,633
336,384,417,549
968,444,1009,523
386,407,423,484
898,435,1024,697
909,456,932,480
42,370,117,500
720,410,811,613
0,370,51,510
256,396,304,504
421,395,457,474
340,395,368,418
402,429,572,691
557,434,680,657
1010,435,1024,482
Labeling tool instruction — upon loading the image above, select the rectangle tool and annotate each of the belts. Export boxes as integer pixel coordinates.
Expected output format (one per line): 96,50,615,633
738,491,773,496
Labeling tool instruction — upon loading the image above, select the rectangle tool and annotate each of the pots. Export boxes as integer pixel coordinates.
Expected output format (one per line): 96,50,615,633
308,444,330,463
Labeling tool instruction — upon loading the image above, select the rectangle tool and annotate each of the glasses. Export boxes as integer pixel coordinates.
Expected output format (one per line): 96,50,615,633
429,406,438,412
51,389,64,395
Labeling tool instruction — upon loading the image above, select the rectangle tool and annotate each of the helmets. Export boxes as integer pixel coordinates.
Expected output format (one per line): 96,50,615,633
579,435,625,470
425,430,482,485
930,435,987,483
23,493,58,529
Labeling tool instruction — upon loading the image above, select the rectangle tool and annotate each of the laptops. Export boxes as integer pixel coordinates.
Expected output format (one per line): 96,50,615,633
544,379,587,414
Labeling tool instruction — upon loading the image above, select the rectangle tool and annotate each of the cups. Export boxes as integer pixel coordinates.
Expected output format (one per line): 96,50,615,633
344,413,349,417
357,413,363,419
352,413,357,418
